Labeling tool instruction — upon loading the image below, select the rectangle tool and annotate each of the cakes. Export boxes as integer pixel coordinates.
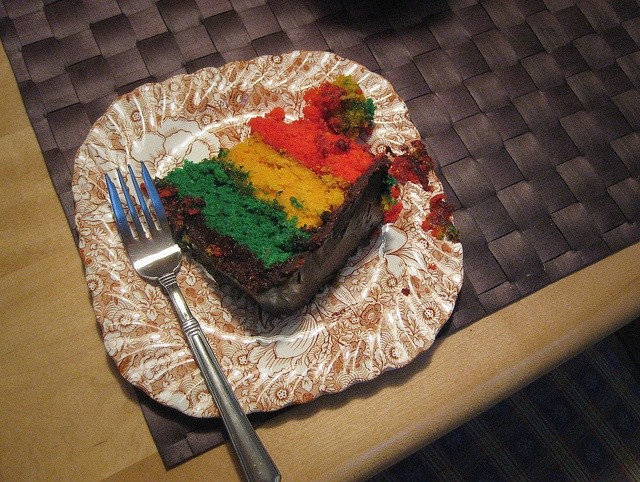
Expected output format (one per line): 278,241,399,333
135,73,395,317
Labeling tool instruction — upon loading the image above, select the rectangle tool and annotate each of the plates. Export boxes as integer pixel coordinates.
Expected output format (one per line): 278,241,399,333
72,50,464,418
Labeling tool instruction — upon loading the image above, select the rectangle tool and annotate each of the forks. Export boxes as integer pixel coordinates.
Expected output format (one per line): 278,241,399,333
105,161,282,482
